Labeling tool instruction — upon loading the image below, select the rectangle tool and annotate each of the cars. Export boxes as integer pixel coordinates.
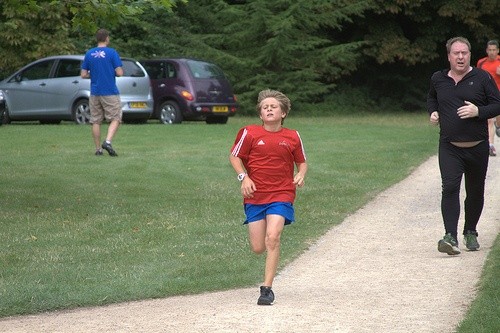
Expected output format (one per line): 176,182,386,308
0,55,153,124
138,58,237,124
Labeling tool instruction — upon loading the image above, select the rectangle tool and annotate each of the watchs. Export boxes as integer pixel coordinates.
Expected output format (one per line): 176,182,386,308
238,173,248,182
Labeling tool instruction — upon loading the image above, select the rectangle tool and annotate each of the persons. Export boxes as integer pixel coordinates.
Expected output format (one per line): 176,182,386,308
427,36,499,255
81,28,124,157
476,40,500,157
229,89,307,306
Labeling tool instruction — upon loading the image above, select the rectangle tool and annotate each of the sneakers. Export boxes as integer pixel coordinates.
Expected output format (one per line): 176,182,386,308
494,121,500,137
96,151,102,156
101,141,117,157
463,234,480,250
257,286,276,305
489,146,496,156
438,235,461,255
